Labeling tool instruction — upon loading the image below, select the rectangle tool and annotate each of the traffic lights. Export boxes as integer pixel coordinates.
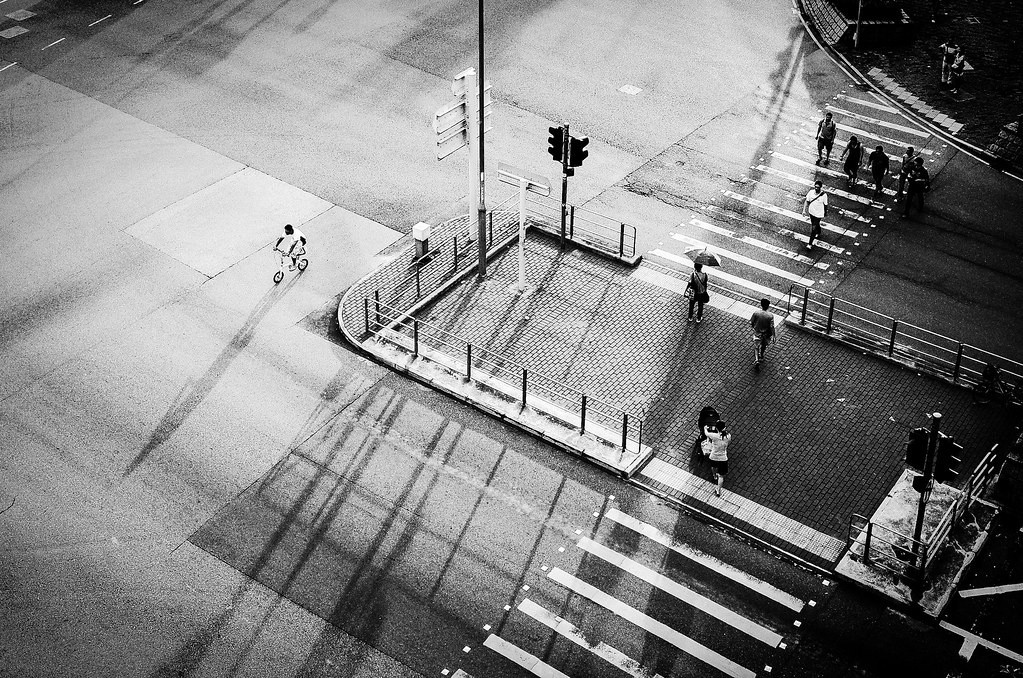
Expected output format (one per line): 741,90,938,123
570,136,589,167
548,125,564,160
934,434,964,485
905,427,932,472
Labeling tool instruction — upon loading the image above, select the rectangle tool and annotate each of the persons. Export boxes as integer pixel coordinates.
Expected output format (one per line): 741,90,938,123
749,298,777,366
683,263,708,322
938,37,965,94
894,147,930,217
866,146,889,203
815,112,837,164
840,136,864,181
704,420,732,497
273,223,307,272
802,181,828,249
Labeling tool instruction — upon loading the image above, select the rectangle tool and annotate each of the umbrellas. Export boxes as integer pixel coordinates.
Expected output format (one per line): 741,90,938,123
686,245,721,267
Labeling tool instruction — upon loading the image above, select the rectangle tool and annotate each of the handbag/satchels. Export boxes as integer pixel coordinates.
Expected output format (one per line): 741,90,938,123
703,291,709,303
684,282,695,301
701,436,713,455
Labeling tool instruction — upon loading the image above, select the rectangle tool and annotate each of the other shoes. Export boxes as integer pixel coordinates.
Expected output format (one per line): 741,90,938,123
950,88,958,94
715,487,720,497
816,157,823,163
289,266,296,271
688,316,694,322
824,159,830,165
815,232,822,237
752,360,760,366
696,316,703,323
806,244,812,250
760,356,764,360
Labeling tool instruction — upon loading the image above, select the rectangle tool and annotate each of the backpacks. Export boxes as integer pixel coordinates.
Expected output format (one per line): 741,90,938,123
820,119,836,138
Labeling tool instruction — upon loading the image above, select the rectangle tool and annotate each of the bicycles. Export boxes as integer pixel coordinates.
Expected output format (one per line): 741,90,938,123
273,247,308,283
